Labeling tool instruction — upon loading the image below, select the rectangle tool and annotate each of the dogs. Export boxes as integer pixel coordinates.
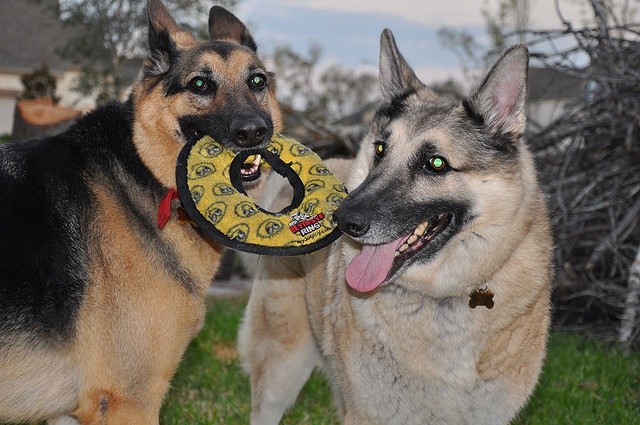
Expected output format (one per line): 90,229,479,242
236,29,554,424
0,0,283,425
